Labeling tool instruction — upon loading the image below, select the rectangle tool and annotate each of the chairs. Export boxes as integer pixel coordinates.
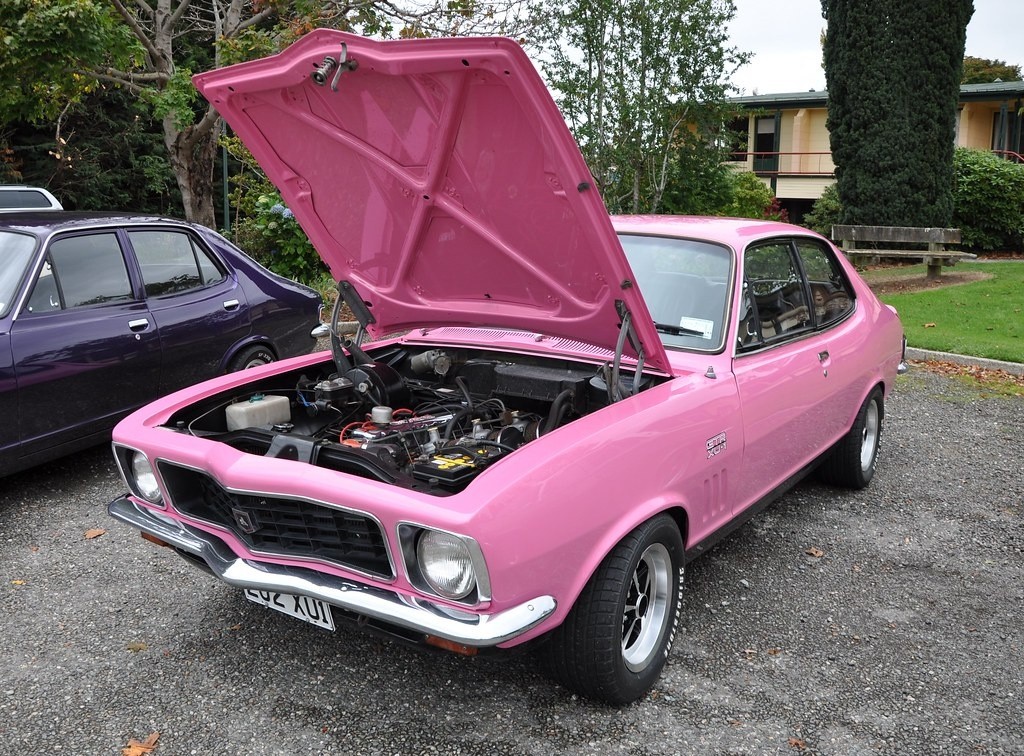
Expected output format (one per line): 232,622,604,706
645,271,754,345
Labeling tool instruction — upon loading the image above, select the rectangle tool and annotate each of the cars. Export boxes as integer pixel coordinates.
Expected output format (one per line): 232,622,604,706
0,207,328,477
104,27,911,705
0,183,65,210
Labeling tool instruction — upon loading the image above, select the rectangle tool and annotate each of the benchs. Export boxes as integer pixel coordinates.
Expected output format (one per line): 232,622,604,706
832,224,977,279
